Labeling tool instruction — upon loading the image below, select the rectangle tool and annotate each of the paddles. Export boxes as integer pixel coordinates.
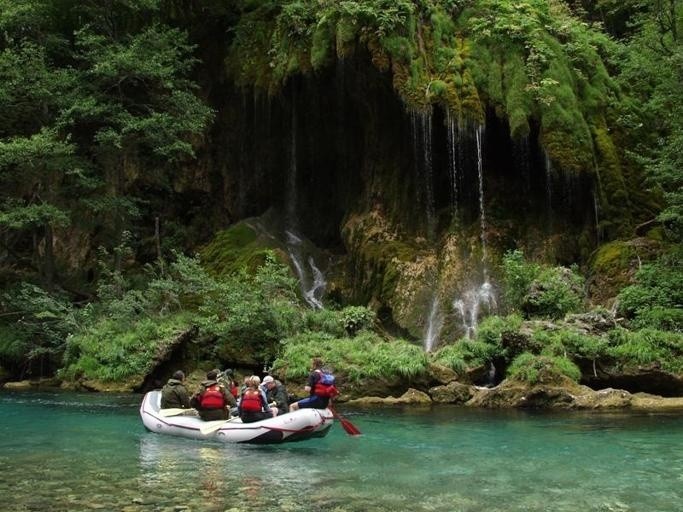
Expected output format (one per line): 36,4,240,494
329,405,360,435
200,401,277,434
159,408,196,417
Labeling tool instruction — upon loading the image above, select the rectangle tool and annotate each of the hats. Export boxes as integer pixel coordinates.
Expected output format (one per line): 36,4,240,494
260,375,274,385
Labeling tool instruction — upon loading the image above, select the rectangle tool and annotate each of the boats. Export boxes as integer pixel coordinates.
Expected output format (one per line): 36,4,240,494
139,389,334,445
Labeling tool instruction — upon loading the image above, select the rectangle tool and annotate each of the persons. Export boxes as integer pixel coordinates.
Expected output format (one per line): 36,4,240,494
161,370,191,410
190,368,289,422
289,358,340,412
